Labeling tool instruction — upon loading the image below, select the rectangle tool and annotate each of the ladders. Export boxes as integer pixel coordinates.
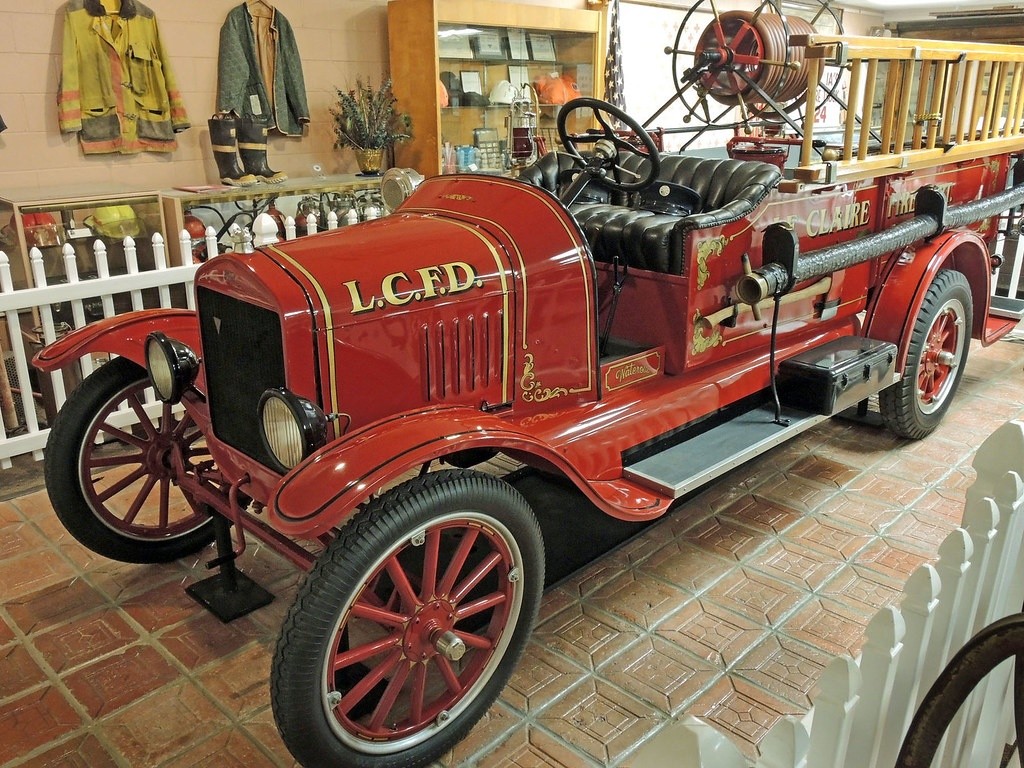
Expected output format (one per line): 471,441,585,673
779,34,1024,196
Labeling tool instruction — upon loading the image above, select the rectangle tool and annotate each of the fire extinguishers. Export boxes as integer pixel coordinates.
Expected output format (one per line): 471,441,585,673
181,189,385,265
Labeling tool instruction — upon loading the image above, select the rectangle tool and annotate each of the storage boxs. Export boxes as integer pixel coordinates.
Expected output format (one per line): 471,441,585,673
777,334,898,416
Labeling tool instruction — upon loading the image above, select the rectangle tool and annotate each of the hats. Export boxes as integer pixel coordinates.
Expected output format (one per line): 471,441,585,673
560,169,607,205
639,181,701,217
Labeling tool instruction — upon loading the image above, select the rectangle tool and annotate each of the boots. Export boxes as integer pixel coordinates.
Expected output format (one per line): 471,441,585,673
238,118,289,183
207,114,256,186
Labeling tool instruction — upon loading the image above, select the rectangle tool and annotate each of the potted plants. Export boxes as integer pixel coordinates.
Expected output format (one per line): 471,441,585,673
328,74,416,178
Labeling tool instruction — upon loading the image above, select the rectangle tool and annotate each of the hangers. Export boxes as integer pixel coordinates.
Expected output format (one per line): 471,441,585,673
99,0,127,15
242,0,281,20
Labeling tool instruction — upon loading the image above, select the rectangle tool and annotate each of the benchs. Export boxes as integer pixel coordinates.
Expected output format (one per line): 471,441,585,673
514,150,782,277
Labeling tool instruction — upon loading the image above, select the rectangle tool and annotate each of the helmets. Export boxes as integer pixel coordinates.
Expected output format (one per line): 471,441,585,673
86,206,146,239
490,81,516,104
10,212,62,247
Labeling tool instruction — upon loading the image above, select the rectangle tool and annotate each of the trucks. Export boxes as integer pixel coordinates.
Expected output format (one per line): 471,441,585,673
32,0,1024,768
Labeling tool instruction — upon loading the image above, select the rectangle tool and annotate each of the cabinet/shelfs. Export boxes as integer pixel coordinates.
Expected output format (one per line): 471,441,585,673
386,1,610,181
159,171,424,265
1,184,174,446
